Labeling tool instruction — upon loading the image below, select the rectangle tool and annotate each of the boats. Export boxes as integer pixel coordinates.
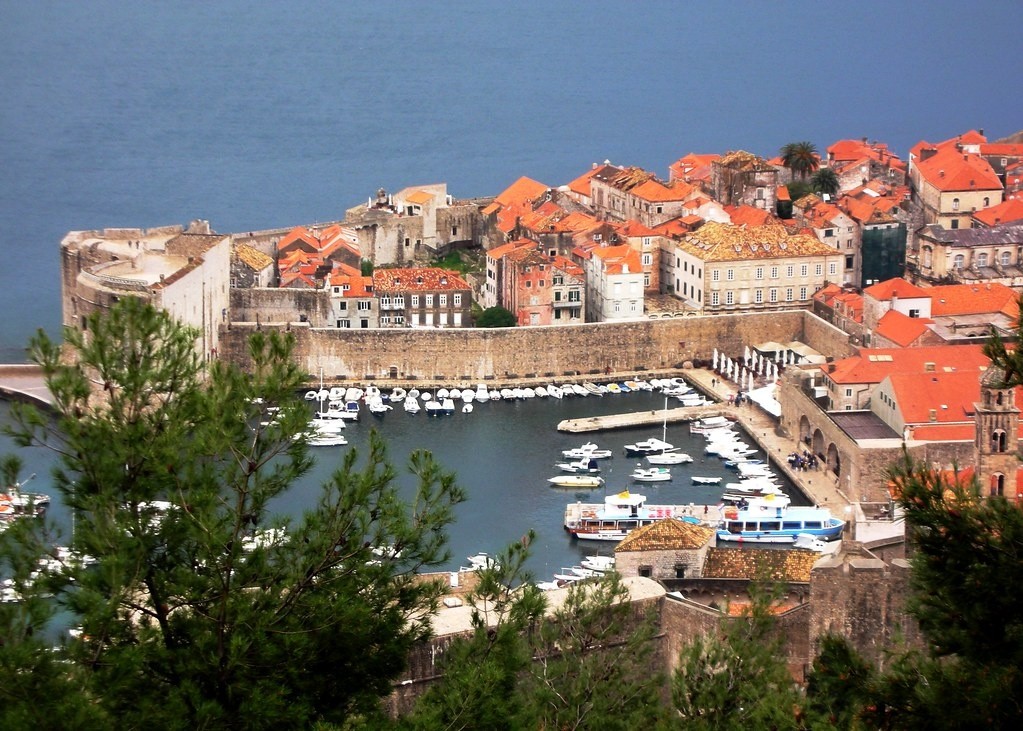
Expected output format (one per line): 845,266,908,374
241,377,849,591
0,475,194,667
